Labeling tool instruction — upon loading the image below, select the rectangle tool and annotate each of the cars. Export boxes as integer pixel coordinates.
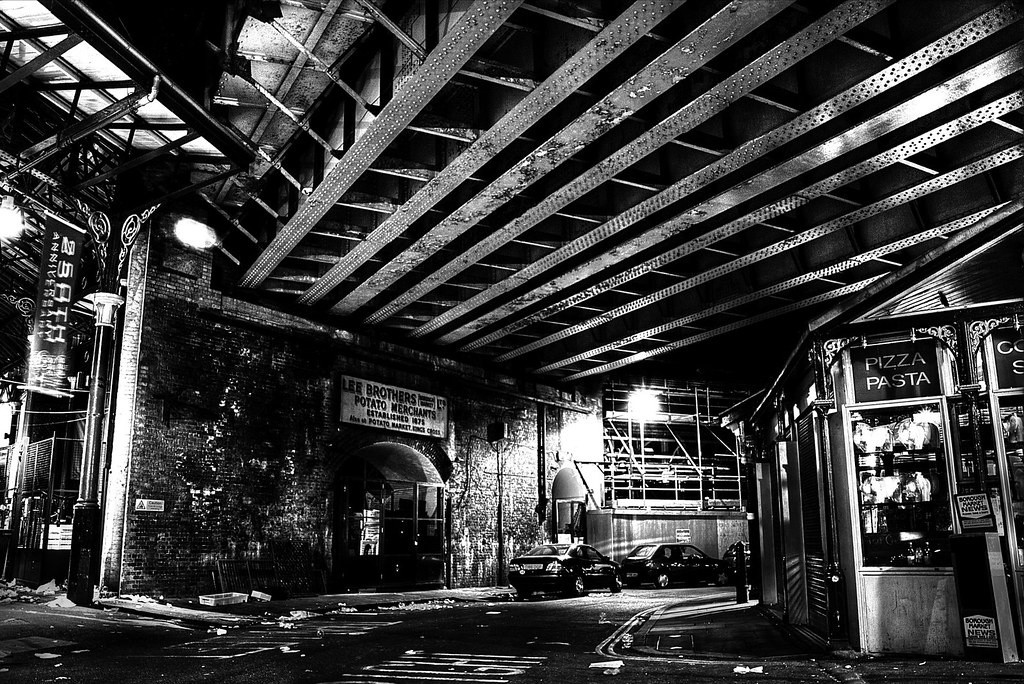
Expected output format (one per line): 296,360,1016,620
723,541,751,584
618,543,729,589
508,544,622,597
